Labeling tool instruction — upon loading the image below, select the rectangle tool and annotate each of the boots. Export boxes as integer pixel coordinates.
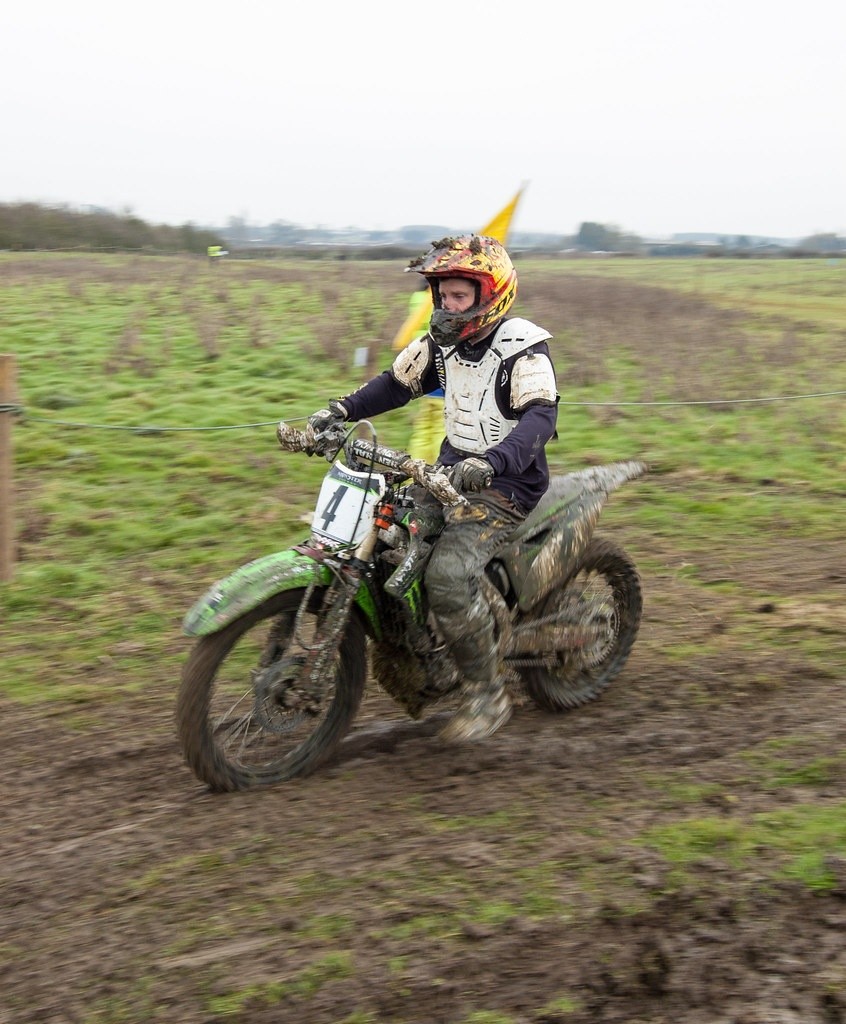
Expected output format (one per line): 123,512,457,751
436,593,513,744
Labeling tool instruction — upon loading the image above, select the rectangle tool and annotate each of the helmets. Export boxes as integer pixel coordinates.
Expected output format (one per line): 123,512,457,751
404,235,521,346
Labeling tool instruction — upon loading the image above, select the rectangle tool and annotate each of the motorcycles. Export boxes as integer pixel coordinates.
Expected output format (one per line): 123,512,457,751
172,402,650,794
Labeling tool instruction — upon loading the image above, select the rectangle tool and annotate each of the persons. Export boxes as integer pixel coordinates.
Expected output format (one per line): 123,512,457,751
208,244,222,264
408,279,446,464
305,236,558,743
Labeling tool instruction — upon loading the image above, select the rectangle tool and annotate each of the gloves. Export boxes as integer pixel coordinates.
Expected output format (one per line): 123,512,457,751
450,458,495,494
303,400,347,457
